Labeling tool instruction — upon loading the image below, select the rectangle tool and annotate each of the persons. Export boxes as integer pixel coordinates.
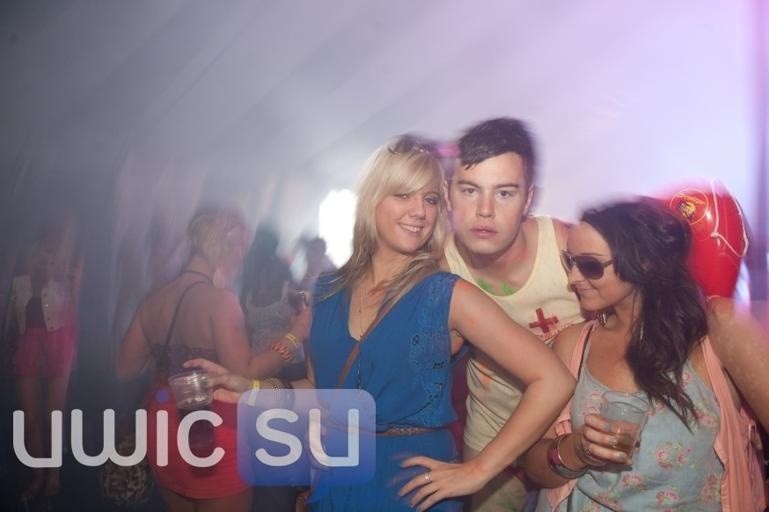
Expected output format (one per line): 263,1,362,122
113,200,308,510
244,222,337,378
181,134,576,511
7,235,83,505
525,197,769,512
436,119,584,511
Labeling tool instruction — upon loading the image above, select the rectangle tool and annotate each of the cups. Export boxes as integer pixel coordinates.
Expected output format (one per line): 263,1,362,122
598,391,650,466
169,371,215,435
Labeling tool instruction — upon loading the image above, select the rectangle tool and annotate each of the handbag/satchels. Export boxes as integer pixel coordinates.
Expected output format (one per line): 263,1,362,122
100,437,154,507
697,292,769,512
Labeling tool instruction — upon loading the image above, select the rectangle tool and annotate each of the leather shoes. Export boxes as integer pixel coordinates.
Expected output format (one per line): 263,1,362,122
18,471,63,503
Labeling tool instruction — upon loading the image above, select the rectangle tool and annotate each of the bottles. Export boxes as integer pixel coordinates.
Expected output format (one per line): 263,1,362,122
294,290,307,315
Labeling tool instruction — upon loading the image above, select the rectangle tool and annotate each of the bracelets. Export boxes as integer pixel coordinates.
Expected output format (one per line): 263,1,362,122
266,373,286,410
247,376,260,411
281,375,296,414
546,432,590,480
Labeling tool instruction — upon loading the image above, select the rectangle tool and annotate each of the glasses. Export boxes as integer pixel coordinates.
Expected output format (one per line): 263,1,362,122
386,130,445,164
558,249,616,281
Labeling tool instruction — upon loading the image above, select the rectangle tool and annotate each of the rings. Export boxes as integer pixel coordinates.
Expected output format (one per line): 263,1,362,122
424,471,432,482
